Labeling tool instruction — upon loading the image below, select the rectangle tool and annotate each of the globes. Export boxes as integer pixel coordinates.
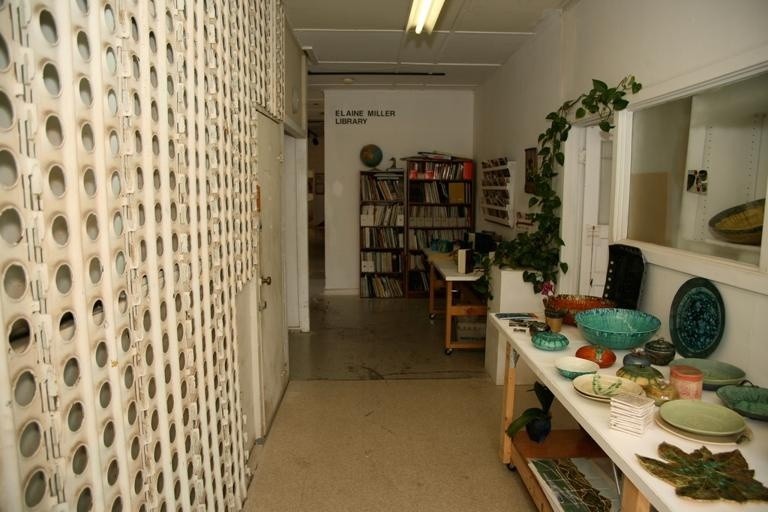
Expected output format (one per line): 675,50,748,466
360,144,384,172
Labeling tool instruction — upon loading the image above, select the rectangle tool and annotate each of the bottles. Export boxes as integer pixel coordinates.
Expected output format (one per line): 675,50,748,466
530,321,551,338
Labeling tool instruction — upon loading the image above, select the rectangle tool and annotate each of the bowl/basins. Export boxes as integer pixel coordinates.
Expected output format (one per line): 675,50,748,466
541,294,617,325
556,356,600,380
709,197,764,245
574,307,662,349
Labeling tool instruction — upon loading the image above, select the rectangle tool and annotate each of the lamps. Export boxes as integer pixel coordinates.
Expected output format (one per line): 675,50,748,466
404,0,445,36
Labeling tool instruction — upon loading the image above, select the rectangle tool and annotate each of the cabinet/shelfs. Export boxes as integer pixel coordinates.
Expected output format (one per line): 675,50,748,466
358,171,406,299
481,161,514,229
400,157,476,299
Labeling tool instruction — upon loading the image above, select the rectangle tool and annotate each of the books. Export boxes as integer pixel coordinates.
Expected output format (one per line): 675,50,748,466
361,152,514,299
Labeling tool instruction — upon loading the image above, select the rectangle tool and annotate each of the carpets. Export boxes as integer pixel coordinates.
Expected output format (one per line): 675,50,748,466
241,379,536,512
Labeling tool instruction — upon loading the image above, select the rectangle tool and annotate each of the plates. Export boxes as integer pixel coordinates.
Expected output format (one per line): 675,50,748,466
575,338,768,444
668,277,726,359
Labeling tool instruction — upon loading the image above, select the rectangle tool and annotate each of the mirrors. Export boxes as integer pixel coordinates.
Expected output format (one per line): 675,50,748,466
610,48,768,296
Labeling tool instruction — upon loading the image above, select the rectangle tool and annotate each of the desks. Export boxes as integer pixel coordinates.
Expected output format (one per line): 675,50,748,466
424,247,491,351
488,311,768,512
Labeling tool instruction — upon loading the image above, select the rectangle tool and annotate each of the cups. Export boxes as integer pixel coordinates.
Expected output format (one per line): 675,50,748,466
546,317,564,333
431,238,455,253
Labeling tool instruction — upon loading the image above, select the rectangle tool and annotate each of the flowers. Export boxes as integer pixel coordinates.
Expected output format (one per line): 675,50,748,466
534,270,556,298
